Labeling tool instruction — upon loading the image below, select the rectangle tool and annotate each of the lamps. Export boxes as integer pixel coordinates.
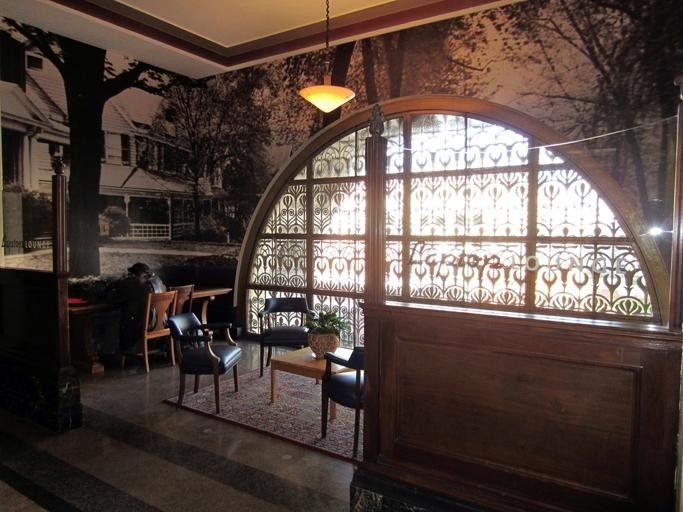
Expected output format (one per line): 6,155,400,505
298,0,355,114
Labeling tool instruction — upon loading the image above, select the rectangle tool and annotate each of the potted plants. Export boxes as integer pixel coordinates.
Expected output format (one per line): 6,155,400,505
304,306,351,359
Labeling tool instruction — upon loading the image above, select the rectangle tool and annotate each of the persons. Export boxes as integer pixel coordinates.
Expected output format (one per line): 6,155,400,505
100,261,169,365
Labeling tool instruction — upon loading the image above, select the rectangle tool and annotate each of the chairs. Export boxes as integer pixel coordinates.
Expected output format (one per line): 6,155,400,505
167,312,242,414
321,347,364,457
258,298,315,377
167,284,194,366
118,284,180,374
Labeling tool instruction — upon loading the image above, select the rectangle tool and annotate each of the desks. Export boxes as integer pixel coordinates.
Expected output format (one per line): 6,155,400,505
68,288,233,375
269,347,355,424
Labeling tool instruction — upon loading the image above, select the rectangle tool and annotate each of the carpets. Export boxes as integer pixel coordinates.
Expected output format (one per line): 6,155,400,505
162,365,365,465
